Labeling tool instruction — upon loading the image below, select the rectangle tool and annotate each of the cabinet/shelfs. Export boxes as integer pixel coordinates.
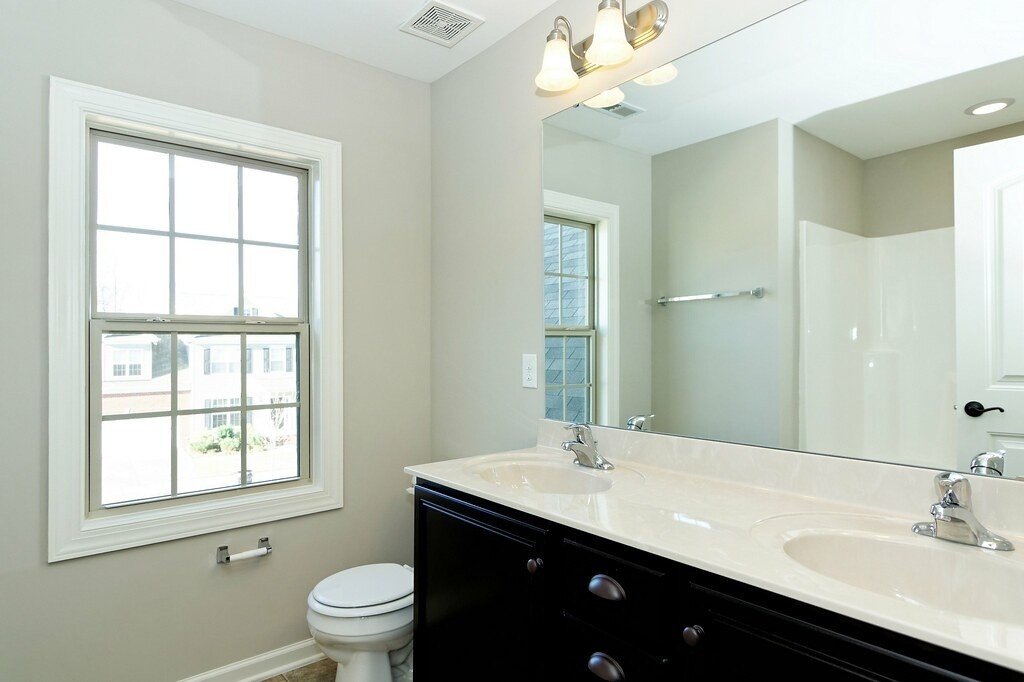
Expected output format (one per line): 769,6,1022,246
403,444,1024,682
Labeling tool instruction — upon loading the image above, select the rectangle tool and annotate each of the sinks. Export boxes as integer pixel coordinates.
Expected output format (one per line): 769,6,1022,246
782,531,1024,629
477,463,613,495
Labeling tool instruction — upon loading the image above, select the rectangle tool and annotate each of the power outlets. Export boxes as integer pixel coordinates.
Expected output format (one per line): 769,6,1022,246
522,354,539,389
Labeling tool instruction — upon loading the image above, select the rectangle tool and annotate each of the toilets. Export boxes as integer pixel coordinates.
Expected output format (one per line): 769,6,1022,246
306,487,412,681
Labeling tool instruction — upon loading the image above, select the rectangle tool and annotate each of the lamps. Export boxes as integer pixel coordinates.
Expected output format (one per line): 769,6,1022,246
582,62,679,111
534,0,669,92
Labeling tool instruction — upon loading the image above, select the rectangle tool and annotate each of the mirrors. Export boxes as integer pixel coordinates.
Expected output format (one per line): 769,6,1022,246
523,0,1024,482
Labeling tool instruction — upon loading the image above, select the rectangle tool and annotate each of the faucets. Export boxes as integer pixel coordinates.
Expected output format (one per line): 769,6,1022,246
971,447,1008,478
561,423,614,470
909,472,1017,551
626,413,655,432
